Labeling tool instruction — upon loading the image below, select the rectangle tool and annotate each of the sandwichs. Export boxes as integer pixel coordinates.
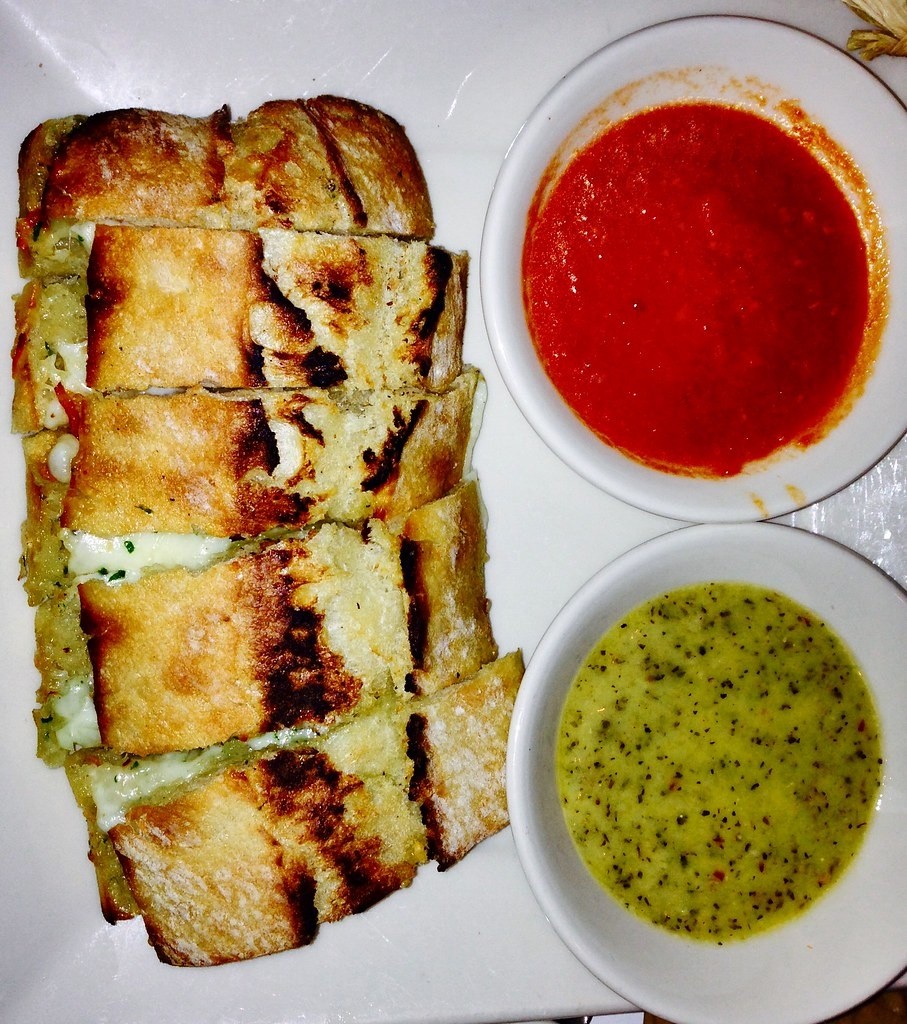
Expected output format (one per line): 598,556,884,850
20,99,527,969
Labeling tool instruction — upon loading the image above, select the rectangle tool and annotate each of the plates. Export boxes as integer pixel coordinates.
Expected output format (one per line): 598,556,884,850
0,0,907,1024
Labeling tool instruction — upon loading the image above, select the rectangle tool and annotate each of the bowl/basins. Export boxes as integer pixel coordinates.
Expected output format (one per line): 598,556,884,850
505,522,907,1024
478,14,907,522
